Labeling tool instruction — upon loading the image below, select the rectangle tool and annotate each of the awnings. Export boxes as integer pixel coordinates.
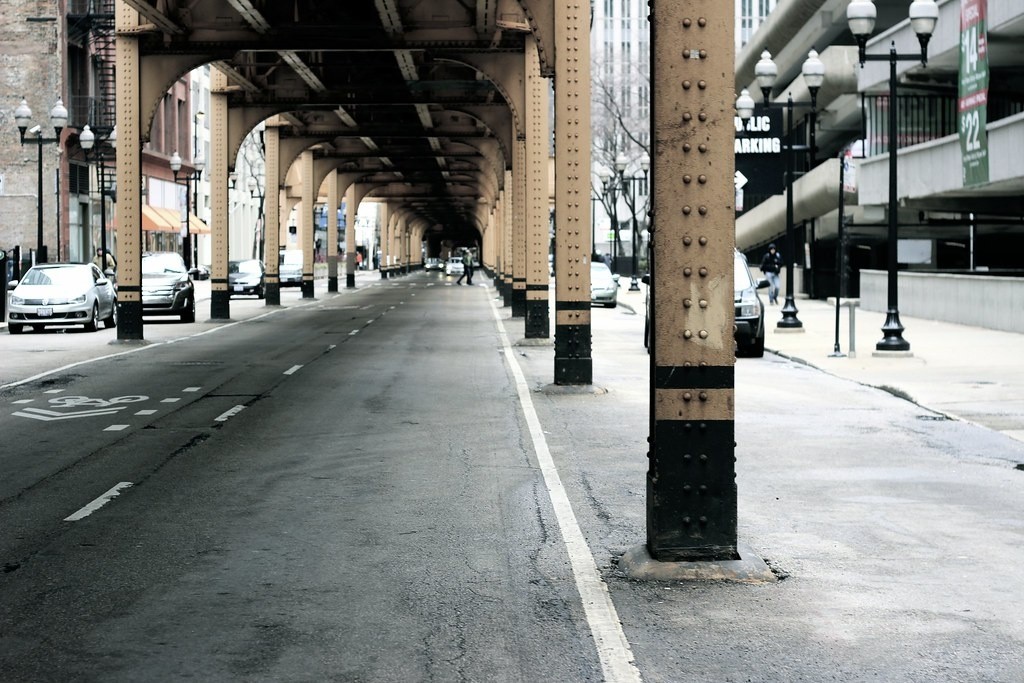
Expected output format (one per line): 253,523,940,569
114,204,210,234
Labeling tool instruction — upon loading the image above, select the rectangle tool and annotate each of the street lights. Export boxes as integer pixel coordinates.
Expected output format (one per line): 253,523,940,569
622,163,646,291
248,178,265,260
845,1,943,357
80,123,117,278
14,95,68,263
590,162,610,261
170,148,206,281
754,46,825,334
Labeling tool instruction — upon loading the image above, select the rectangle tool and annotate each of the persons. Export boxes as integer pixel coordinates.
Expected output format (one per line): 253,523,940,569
93,248,117,282
356,251,362,271
760,244,782,305
456,249,474,285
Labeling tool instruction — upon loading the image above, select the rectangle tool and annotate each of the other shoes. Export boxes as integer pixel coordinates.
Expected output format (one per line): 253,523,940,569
456,281,461,285
774,297,778,304
467,281,473,285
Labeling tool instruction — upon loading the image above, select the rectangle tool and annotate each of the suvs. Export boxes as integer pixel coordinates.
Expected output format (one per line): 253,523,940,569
643,247,770,358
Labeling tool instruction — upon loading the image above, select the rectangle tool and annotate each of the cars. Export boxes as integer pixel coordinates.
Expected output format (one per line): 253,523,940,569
104,250,201,325
424,257,445,272
279,249,304,287
6,261,118,334
444,257,465,276
228,260,265,299
590,261,621,308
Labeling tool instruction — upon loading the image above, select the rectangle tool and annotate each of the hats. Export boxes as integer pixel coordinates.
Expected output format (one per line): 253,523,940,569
769,244,776,250
